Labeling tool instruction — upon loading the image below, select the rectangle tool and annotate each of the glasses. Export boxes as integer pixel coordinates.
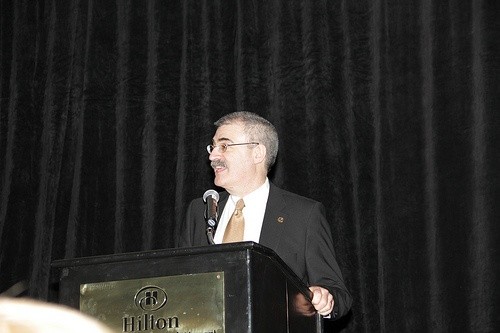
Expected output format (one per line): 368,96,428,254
206,142,259,155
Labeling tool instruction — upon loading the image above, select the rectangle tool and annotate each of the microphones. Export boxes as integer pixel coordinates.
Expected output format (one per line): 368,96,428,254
203,189,220,226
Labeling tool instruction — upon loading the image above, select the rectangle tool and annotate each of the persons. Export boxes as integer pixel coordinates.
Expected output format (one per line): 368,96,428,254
179,112,353,321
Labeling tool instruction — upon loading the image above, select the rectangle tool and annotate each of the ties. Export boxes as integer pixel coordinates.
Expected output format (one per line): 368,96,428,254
222,199,246,244
